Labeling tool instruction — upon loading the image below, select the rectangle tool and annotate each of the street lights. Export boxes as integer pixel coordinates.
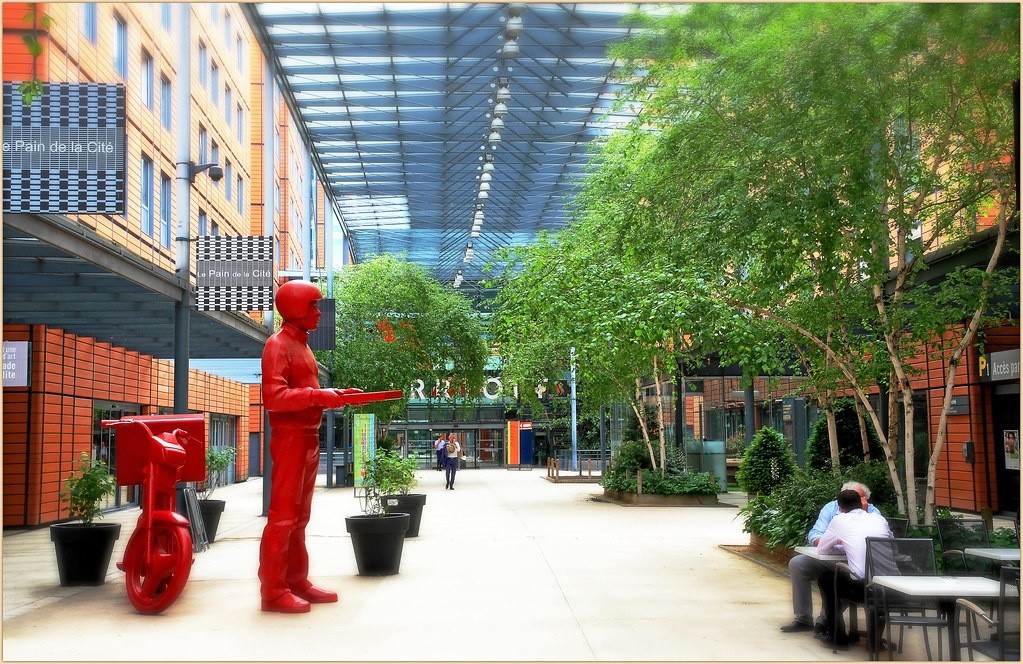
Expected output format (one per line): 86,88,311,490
174,163,224,513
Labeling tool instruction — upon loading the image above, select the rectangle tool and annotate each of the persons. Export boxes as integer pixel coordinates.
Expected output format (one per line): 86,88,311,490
257,280,371,613
435,436,445,471
1006,432,1018,454
780,481,881,638
440,434,460,490
818,490,901,652
94,442,107,463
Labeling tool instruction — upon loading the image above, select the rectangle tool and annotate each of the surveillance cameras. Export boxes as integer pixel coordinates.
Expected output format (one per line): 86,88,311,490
209,166,223,182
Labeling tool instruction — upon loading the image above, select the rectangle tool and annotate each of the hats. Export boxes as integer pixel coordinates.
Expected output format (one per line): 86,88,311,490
447,444,455,453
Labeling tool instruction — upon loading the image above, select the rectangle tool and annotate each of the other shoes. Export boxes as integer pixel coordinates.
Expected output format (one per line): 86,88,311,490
445,483,449,489
819,636,849,651
779,621,813,631
450,487,454,490
439,469,442,471
866,642,886,652
813,623,827,639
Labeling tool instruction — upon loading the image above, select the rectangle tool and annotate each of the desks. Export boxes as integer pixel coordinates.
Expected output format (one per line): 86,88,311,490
872,575,1019,661
793,546,859,645
964,548,1020,661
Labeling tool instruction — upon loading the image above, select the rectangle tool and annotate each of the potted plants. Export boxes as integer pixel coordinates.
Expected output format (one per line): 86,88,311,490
49,451,121,587
179,444,245,544
380,442,427,538
344,446,410,578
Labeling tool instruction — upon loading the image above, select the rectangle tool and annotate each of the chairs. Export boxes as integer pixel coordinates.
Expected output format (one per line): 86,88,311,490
833,515,1020,661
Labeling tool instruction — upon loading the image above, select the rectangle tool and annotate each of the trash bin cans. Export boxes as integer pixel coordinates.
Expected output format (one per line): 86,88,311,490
334,465,347,487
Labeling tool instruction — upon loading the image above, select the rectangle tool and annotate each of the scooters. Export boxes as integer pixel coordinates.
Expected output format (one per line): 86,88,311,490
100,412,205,615
